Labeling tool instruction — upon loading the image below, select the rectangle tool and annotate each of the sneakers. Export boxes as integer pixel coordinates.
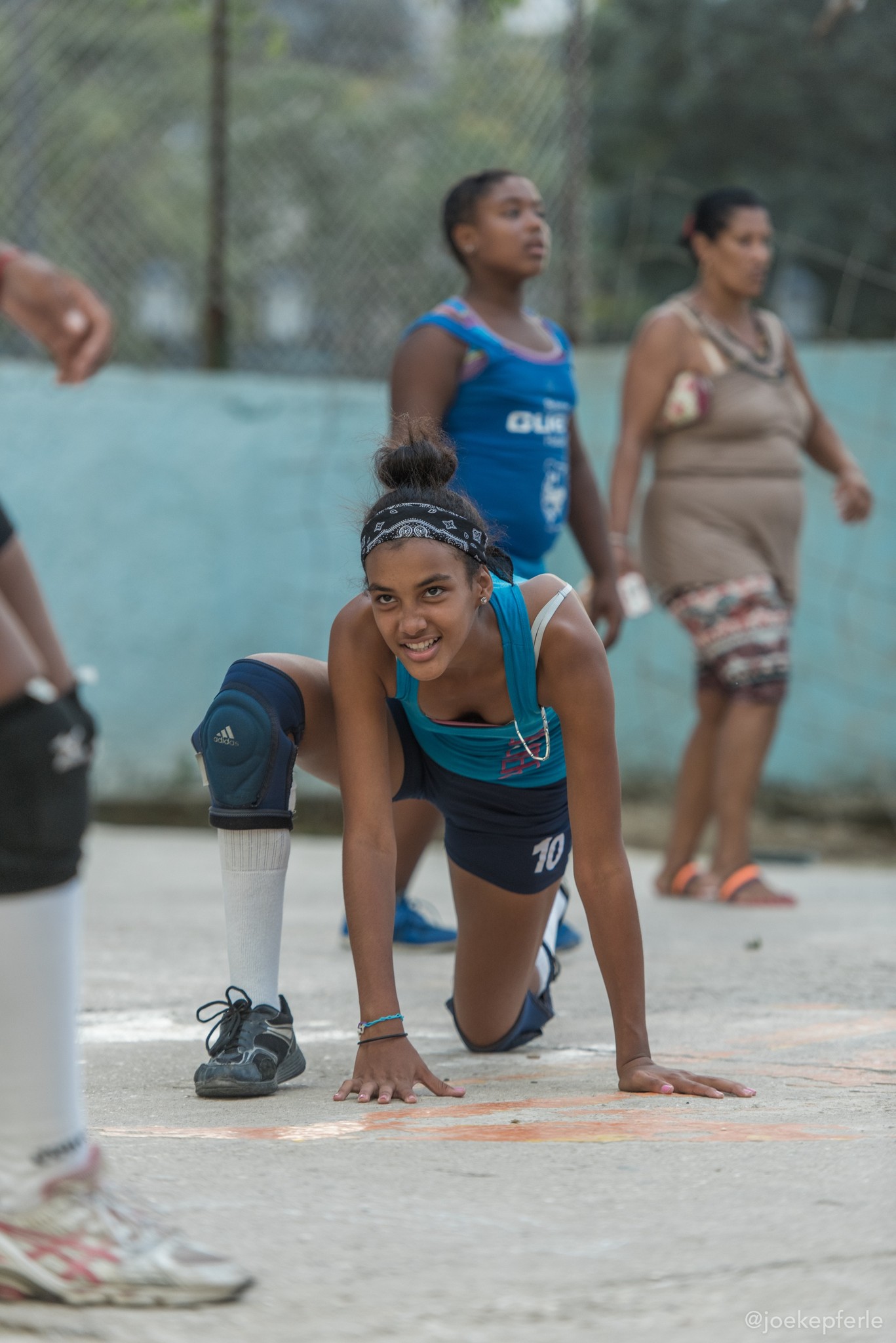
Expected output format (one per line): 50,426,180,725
339,893,457,953
1,1137,257,1307
192,986,306,1096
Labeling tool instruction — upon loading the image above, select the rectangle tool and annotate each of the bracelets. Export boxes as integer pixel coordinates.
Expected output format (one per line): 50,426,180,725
606,528,627,544
354,1013,404,1036
356,1033,407,1042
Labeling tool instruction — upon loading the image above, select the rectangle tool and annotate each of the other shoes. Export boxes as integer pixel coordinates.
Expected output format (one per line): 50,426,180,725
656,860,714,901
714,860,794,907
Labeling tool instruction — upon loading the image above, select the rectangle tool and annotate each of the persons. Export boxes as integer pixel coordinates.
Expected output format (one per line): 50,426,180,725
0,244,257,1310
607,191,874,906
340,169,625,951
190,420,755,1103
